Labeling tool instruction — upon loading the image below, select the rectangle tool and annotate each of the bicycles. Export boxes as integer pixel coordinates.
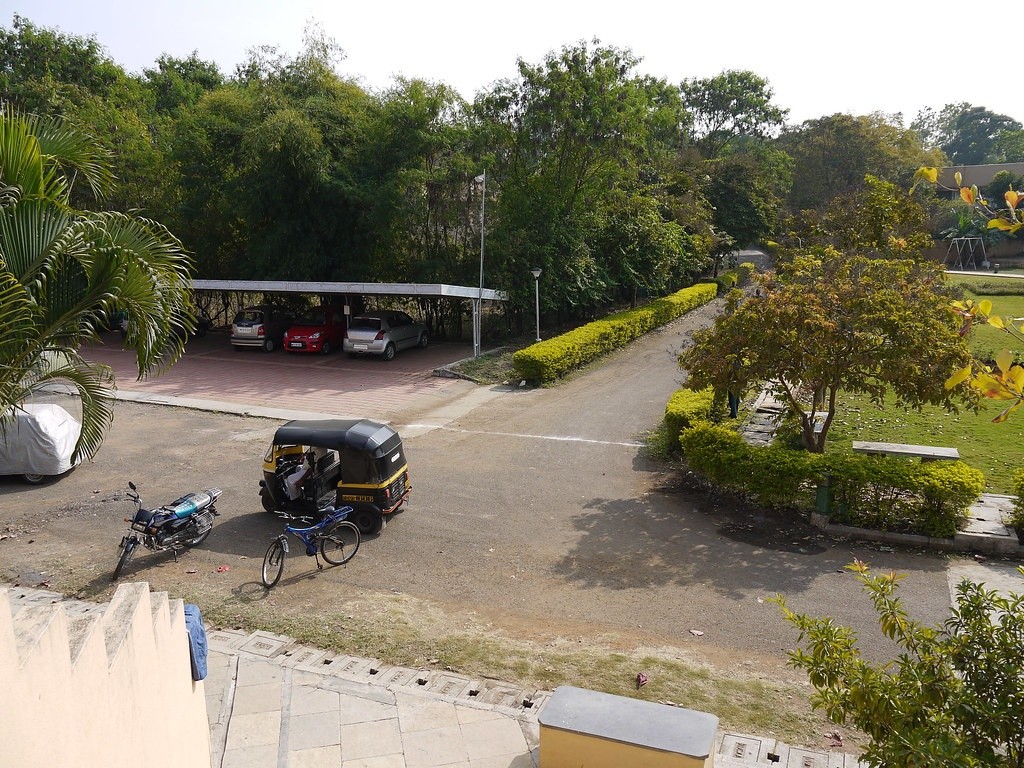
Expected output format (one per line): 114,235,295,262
261,506,361,588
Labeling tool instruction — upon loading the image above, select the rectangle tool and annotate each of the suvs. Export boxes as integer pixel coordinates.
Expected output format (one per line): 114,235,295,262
343,310,429,361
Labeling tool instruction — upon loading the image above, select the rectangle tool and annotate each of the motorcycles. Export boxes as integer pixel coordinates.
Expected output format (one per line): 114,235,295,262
112,480,222,580
257,419,413,534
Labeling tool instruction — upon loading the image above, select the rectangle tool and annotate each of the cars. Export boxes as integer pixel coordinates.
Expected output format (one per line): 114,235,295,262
122,310,207,342
0,404,87,484
283,305,346,354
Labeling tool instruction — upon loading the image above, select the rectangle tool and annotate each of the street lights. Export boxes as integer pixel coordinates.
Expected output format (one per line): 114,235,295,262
474,169,487,357
531,267,544,341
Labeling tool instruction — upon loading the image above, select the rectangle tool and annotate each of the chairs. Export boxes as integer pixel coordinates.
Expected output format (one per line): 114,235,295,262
318,451,334,471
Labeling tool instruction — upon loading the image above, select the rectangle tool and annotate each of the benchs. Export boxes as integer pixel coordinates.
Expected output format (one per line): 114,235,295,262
852,441,960,464
799,412,829,447
538,685,719,768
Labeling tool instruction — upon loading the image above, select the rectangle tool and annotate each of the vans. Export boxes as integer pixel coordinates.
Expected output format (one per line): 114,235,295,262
231,306,302,352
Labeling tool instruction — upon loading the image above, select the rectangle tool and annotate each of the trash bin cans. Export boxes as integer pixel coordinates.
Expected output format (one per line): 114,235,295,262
994,264,999,273
816,470,834,513
